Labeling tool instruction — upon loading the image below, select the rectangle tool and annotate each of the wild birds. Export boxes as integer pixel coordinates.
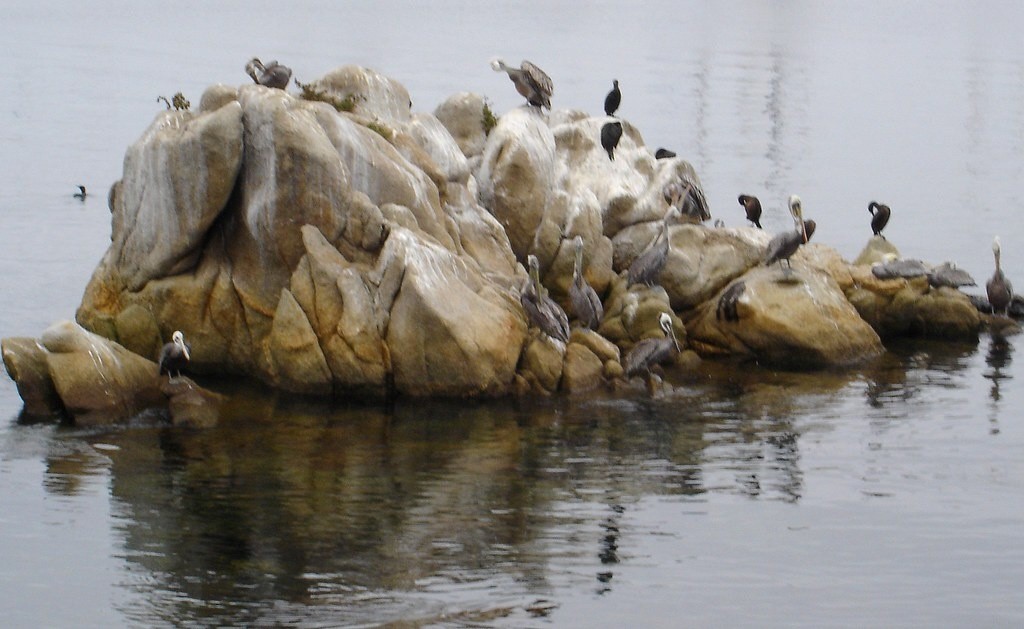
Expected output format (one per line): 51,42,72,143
245,58,292,90
986,237,1014,317
158,330,191,384
519,235,605,344
491,58,554,112
623,312,681,383
626,205,682,290
767,194,816,281
738,194,762,229
868,202,891,235
603,80,622,117
600,121,623,161
74,184,87,197
